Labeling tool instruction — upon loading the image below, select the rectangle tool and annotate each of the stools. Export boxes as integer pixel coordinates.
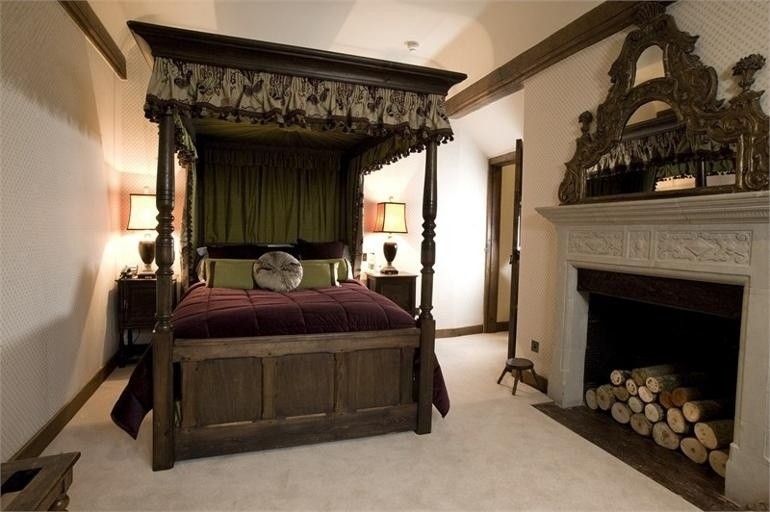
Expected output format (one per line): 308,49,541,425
496,359,546,396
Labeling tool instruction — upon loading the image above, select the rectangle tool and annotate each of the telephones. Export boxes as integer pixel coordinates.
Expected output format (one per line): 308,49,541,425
122,264,138,278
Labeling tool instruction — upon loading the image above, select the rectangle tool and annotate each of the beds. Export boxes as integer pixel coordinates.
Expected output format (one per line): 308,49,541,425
127,20,467,471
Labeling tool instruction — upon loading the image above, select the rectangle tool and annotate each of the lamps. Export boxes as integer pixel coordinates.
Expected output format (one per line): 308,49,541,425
126,193,157,278
372,202,409,274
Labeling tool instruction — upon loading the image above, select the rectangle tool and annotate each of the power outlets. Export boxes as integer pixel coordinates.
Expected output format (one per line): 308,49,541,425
530,340,541,354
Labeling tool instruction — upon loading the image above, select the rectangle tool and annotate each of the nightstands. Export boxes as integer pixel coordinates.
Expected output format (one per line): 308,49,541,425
118,276,177,368
366,272,419,319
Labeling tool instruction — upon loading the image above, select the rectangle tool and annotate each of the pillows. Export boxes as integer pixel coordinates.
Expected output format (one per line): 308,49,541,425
205,258,253,290
194,248,208,284
298,263,331,289
254,251,305,292
330,261,342,286
299,258,351,283
